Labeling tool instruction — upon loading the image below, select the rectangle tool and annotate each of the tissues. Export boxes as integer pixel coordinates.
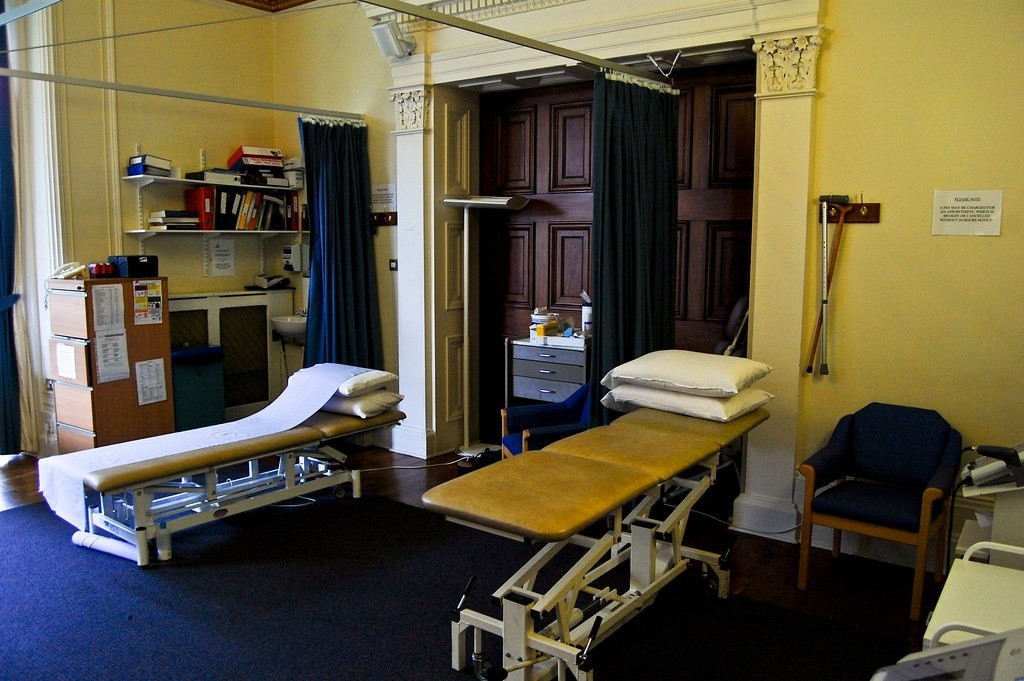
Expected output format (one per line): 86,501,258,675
569,328,592,347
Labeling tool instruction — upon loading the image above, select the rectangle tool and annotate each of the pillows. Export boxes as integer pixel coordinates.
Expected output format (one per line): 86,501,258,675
598,386,774,423
321,390,403,420
290,363,396,396
602,350,771,398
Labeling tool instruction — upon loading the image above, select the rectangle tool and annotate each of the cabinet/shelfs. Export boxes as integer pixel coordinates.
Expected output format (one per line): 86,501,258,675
121,175,301,237
505,336,591,408
46,279,175,454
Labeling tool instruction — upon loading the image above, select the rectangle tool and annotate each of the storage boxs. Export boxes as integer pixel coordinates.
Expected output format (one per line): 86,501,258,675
528,325,558,340
108,256,119,278
118,255,158,278
530,313,558,324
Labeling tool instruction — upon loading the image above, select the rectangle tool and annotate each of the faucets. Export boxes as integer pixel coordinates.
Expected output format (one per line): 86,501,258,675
296,308,307,317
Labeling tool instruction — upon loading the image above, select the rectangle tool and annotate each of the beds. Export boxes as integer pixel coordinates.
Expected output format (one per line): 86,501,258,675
39,410,406,566
427,410,771,681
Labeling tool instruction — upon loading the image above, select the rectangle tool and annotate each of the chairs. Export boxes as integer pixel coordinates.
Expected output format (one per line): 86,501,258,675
500,386,600,458
796,402,963,623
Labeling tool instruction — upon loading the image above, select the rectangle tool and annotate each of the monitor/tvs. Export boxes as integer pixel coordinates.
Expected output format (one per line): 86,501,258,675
971,441,1024,481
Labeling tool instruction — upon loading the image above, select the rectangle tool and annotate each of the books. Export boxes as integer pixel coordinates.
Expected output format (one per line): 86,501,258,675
127,153,300,232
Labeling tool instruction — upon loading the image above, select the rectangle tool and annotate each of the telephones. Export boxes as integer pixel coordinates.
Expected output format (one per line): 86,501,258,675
50,261,87,279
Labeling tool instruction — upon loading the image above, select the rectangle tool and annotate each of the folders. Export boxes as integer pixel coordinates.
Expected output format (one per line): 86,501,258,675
184,145,289,187
126,163,172,177
183,186,299,230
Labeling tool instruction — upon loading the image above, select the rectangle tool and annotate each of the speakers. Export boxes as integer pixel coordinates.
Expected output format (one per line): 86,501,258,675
370,20,406,56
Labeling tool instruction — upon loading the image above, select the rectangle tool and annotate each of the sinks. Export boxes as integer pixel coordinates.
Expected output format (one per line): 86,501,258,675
533,336,585,349
270,316,307,337
